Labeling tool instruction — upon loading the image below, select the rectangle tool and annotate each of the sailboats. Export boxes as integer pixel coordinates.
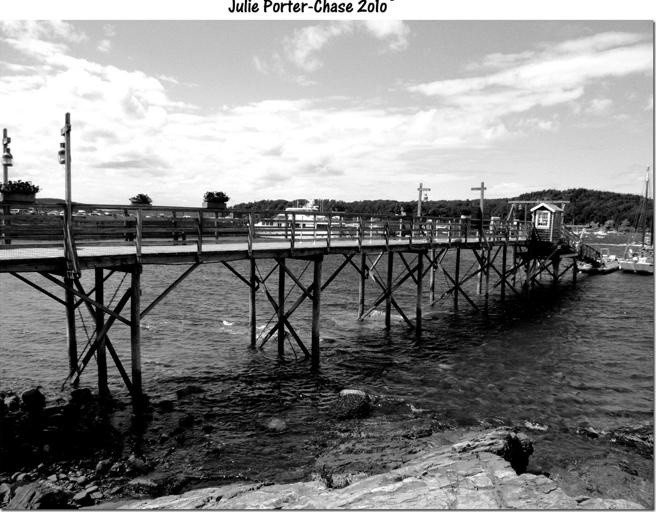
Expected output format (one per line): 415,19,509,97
618,166,654,274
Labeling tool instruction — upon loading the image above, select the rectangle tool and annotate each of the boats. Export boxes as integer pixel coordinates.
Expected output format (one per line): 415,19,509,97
246,204,351,242
576,248,620,275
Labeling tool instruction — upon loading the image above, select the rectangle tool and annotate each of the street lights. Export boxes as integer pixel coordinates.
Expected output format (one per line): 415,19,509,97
471,182,486,236
1,128,13,245
57,113,73,258
417,182,432,215
